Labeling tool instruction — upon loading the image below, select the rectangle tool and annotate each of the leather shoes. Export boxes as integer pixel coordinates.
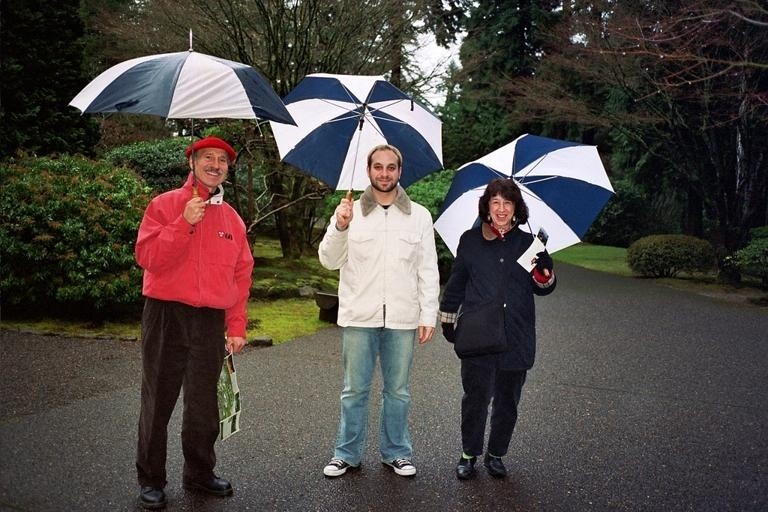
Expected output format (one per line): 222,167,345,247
184,476,233,497
140,487,168,507
455,456,477,481
483,455,508,479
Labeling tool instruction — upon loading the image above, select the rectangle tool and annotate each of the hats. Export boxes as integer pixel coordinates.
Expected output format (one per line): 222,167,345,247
186,136,238,161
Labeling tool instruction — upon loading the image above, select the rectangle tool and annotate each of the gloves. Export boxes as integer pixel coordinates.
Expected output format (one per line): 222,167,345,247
441,322,455,343
536,248,554,275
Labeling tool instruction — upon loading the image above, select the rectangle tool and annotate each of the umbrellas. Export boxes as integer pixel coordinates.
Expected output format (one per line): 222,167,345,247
65,26,300,198
267,70,446,220
433,133,618,277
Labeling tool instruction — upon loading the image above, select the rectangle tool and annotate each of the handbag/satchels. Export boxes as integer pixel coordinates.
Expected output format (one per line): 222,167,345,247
454,230,509,359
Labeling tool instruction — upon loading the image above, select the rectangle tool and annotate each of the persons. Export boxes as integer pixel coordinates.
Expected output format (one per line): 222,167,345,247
133,136,254,512
439,176,558,482
319,144,440,478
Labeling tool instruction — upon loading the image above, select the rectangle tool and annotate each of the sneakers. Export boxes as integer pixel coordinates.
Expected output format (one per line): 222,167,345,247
322,457,351,478
380,458,417,477
